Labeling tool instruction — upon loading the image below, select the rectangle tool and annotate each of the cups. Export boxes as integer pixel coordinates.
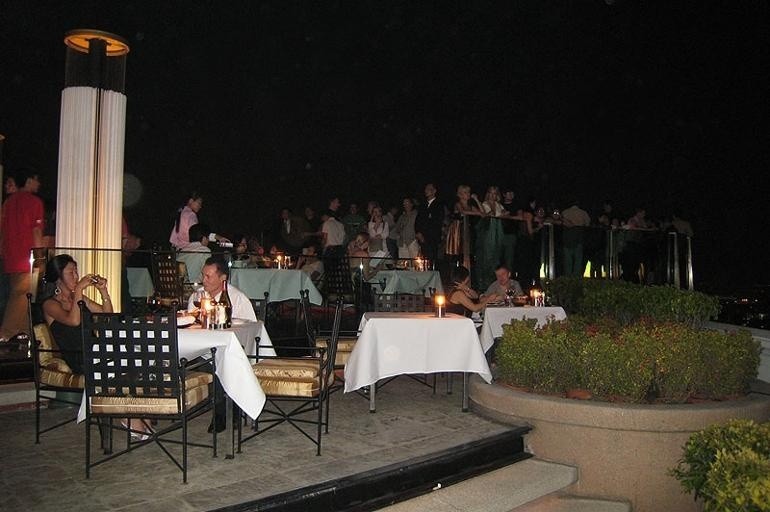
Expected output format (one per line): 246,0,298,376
193,290,205,308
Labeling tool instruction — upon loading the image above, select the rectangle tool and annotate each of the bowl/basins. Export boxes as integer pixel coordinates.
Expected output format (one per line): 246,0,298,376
256,260,275,269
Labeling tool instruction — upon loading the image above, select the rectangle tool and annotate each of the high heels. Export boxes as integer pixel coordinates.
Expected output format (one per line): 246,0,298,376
121,420,156,441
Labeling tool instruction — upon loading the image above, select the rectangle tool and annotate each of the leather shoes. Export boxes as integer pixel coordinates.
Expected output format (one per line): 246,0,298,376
208,415,226,435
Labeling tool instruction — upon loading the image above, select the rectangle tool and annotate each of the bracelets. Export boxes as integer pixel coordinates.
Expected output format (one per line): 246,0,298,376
100,293,111,302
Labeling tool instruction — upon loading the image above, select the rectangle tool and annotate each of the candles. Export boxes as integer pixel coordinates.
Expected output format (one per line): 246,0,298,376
437,295,444,318
535,289,538,307
277,256,281,269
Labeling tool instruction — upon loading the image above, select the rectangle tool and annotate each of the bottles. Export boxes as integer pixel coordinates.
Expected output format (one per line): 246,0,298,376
217,240,245,248
218,280,231,328
544,281,552,306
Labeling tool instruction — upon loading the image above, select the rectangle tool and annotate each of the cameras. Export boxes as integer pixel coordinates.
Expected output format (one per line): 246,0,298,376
88,274,98,286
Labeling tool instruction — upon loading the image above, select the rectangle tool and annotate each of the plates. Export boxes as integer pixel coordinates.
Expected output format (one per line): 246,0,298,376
122,316,196,327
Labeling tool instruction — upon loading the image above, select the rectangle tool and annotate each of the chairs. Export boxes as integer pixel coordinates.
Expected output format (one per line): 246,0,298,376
430,286,438,308
146,240,196,307
300,289,378,400
373,287,437,394
248,292,270,427
25,292,136,453
320,246,371,335
236,296,344,455
78,300,217,482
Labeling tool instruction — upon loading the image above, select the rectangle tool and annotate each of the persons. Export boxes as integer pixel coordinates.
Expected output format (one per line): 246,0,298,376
177,224,213,292
187,257,258,433
476,186,510,264
446,186,485,283
551,208,570,225
382,204,400,265
516,197,544,286
170,193,231,255
500,187,523,262
321,197,344,222
345,203,368,240
593,203,700,281
479,264,524,320
320,210,345,263
414,182,445,288
41,255,158,440
231,234,265,268
279,207,302,262
4,172,20,203
368,207,389,255
0,166,54,343
536,205,550,223
445,266,500,335
280,242,325,314
562,197,591,276
343,233,383,303
396,198,420,268
368,200,380,223
304,207,324,231
